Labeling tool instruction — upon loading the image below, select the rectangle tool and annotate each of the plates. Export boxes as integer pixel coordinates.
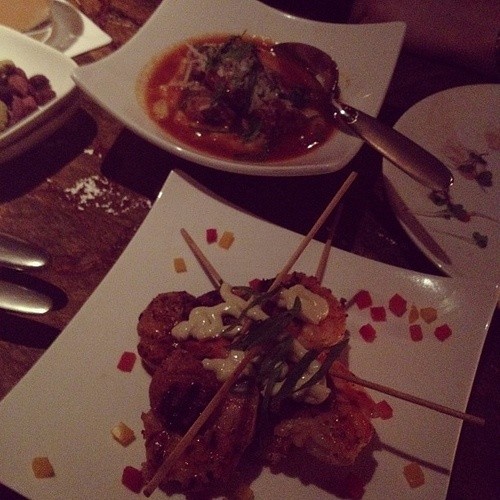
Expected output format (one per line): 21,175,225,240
71,0,406,177
0,24,80,148
0,1,113,58
0,171,500,499
380,82,500,279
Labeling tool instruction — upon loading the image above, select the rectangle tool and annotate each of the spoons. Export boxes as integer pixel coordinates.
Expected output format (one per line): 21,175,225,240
271,42,454,191
